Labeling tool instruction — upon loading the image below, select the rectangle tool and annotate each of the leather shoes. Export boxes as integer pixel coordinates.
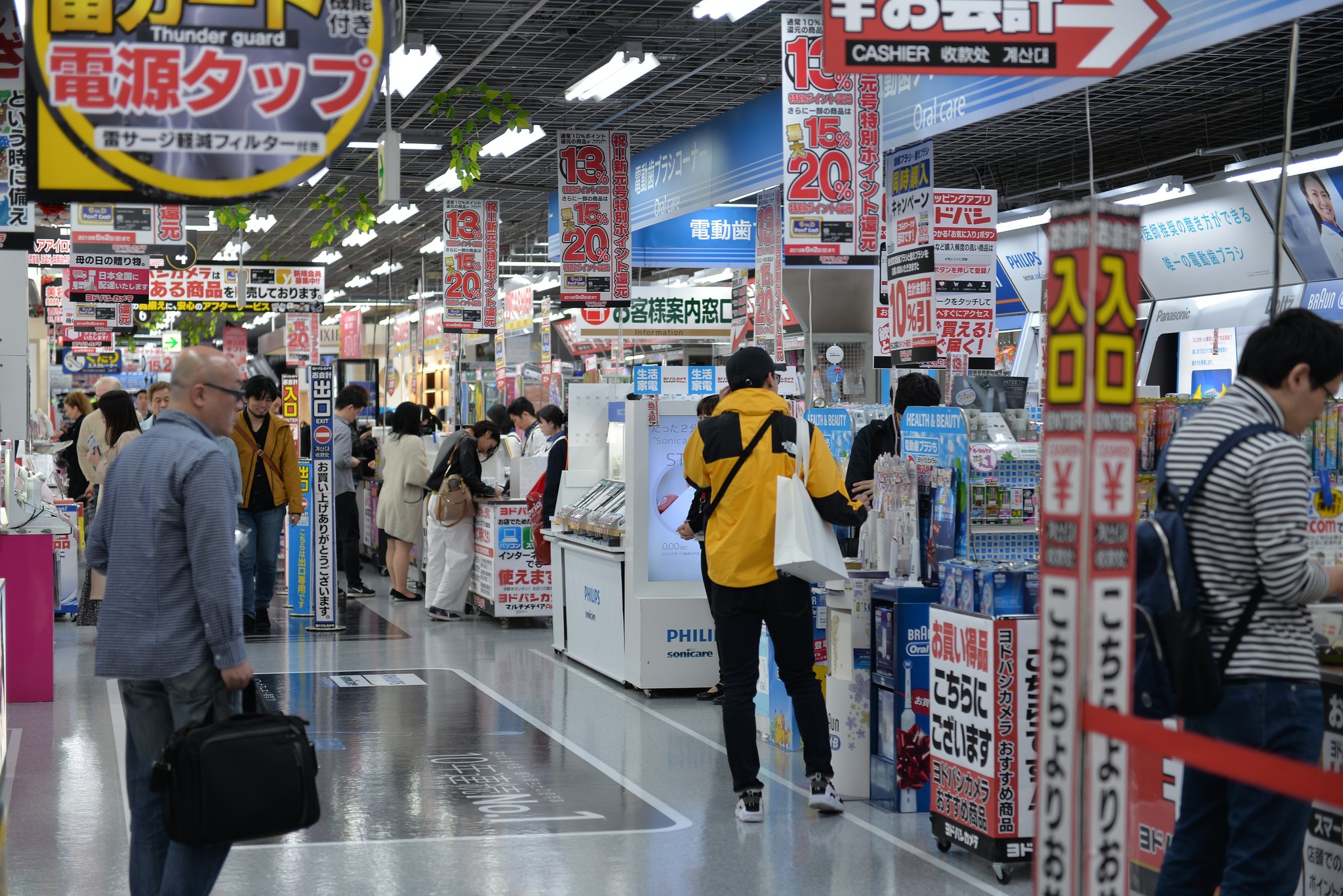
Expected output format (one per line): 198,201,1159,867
712,695,724,705
695,686,724,701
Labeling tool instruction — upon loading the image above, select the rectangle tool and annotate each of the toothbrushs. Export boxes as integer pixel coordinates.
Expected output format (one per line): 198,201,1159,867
860,522,871,570
888,515,903,579
908,517,920,581
899,656,918,813
927,498,934,579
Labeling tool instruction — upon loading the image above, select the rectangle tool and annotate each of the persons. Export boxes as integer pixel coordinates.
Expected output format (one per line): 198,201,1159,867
54,373,567,638
844,372,941,511
676,394,727,706
1117,307,1343,896
83,343,255,896
1297,172,1343,280
681,347,873,824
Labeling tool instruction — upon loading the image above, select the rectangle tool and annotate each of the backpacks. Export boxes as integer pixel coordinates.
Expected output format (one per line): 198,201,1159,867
1135,424,1296,717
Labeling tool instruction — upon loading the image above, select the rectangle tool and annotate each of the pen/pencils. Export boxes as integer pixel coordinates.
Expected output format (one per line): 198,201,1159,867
495,481,498,488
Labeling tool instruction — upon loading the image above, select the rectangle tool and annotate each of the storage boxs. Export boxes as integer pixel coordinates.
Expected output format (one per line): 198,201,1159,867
938,559,1040,617
969,476,1037,525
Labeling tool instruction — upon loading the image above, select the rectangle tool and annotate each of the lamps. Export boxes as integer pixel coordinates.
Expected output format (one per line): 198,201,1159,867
213,208,277,261
380,33,442,99
478,116,547,158
374,198,419,224
335,226,378,247
1082,176,1196,207
308,246,343,265
550,42,661,103
1210,139,1343,184
365,257,403,275
412,232,444,254
344,273,373,288
323,286,346,303
415,159,481,195
996,200,1068,233
678,0,770,23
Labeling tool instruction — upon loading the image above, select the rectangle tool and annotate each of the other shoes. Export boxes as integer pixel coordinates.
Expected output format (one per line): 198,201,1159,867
808,773,844,812
427,606,461,621
243,615,255,635
347,582,376,598
255,608,271,631
430,616,446,621
735,790,765,822
389,588,423,601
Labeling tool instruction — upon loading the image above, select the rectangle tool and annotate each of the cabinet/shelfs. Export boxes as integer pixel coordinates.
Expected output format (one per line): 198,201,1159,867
869,583,943,813
901,406,1042,559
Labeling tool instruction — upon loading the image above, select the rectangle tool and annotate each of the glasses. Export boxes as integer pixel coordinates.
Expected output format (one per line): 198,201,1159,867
775,373,781,385
1322,385,1340,409
202,383,245,402
93,394,101,400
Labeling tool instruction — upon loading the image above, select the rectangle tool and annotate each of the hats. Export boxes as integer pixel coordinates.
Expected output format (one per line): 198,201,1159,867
726,346,786,389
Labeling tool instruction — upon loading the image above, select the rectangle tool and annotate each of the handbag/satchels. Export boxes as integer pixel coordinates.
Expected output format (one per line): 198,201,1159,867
150,677,321,846
772,417,849,584
433,474,476,527
